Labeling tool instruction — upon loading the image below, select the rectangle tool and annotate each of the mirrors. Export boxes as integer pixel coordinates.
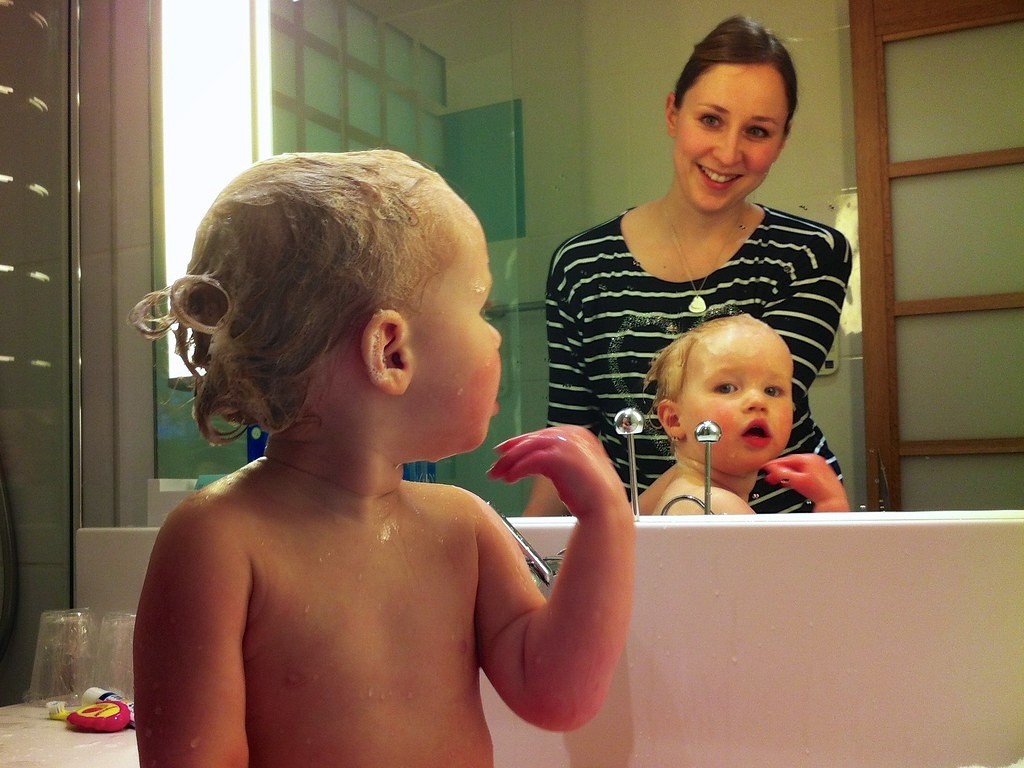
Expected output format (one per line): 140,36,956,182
73,0,1024,527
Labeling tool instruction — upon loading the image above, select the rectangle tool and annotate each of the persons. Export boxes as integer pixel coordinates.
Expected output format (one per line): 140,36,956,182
628,305,850,517
130,146,633,768
621,417,631,432
522,14,852,514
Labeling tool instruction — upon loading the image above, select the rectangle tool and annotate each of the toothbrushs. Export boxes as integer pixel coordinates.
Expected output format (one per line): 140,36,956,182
45,701,71,721
83,687,137,729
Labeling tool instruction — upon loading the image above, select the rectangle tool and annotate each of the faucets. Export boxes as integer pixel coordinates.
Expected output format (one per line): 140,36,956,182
661,494,715,515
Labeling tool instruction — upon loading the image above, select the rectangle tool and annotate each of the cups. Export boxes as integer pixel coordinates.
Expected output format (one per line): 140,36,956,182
27,608,91,706
92,612,136,702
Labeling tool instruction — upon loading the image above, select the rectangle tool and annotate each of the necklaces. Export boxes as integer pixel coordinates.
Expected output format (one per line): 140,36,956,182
660,196,739,313
